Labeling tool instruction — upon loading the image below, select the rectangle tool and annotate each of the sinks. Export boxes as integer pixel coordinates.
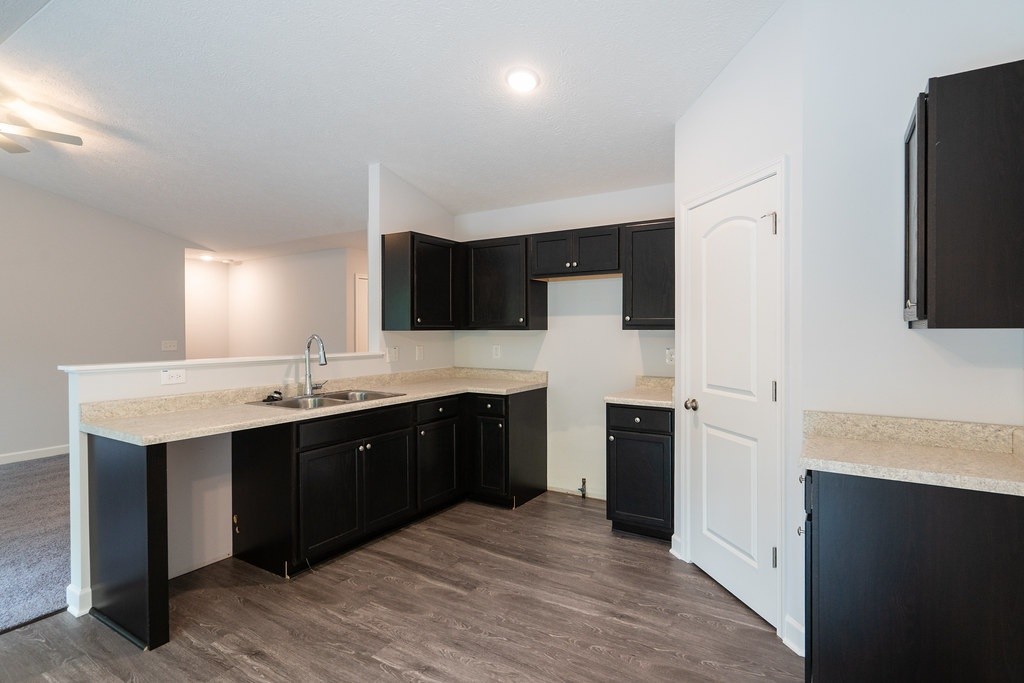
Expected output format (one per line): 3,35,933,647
244,394,348,411
321,388,407,404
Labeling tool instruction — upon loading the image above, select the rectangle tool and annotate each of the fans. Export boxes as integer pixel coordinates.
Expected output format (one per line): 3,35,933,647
0,121,84,155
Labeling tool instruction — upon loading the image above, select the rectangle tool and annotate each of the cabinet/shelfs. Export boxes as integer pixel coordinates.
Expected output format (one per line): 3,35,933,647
416,391,471,523
462,233,549,330
380,230,462,331
795,467,1024,683
471,386,549,510
604,402,674,548
531,223,622,282
231,400,416,582
621,217,675,330
903,58,1024,329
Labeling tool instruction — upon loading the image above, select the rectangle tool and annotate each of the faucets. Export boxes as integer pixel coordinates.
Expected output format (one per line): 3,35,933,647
304,334,328,396
